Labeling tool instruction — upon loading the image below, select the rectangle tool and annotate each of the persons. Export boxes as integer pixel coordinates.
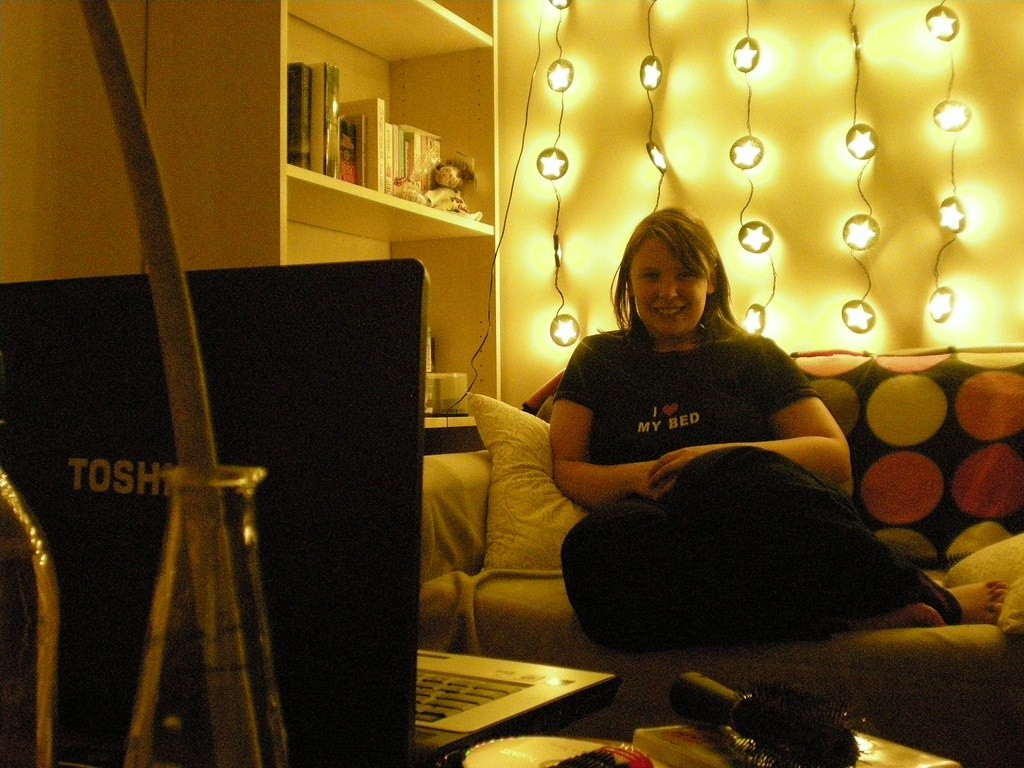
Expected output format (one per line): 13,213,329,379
550,206,1007,650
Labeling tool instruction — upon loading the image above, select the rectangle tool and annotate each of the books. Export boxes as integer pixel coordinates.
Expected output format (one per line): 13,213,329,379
288,61,441,196
634,725,960,768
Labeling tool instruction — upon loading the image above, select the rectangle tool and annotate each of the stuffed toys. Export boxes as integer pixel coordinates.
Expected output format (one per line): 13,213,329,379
397,159,483,221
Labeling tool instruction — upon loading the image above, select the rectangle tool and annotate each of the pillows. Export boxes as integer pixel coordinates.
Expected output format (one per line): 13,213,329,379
466,391,586,581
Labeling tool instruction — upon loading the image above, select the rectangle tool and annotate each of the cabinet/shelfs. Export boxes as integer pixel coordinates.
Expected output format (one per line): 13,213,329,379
136,0,504,464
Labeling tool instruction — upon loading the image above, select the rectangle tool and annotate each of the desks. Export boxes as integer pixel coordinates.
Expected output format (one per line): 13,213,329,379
438,703,958,768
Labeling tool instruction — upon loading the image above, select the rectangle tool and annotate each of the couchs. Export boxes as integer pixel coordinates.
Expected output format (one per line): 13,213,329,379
421,344,1024,768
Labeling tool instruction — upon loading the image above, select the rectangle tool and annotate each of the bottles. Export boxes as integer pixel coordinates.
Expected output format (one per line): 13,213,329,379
123,468,292,768
0,468,58,768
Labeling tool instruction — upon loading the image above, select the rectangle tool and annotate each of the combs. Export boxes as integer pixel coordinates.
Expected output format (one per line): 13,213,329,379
671,671,861,768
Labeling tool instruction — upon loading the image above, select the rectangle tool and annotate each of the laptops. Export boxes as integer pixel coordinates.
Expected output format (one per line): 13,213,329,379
0,258,622,768
